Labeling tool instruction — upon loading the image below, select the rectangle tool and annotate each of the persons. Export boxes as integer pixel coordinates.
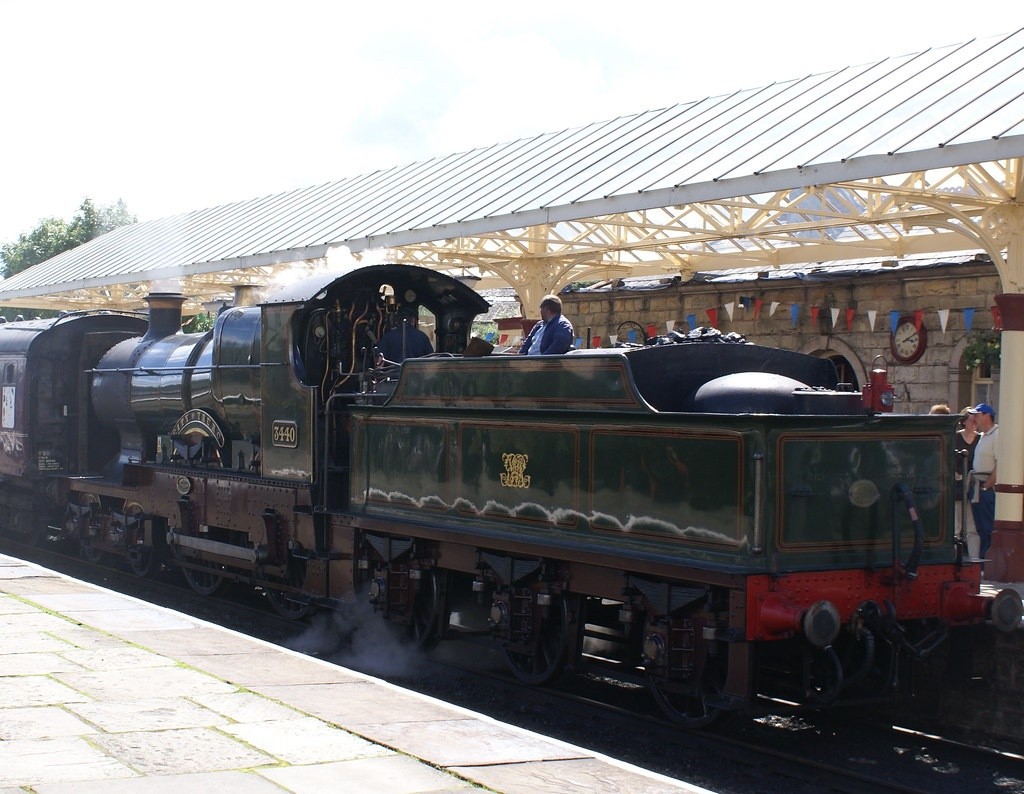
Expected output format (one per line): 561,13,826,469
956,406,980,558
518,295,574,356
379,308,434,378
969,404,998,571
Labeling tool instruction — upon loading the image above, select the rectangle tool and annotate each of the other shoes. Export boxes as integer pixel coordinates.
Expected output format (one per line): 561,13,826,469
980,570,985,576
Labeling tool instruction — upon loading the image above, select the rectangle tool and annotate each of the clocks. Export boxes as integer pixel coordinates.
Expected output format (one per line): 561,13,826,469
890,316,928,365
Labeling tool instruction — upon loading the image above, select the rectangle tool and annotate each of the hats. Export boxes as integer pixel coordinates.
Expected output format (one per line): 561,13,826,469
966,403,996,415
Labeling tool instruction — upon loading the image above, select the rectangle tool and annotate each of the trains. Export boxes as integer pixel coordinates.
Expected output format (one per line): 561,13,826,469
0,262,983,750
